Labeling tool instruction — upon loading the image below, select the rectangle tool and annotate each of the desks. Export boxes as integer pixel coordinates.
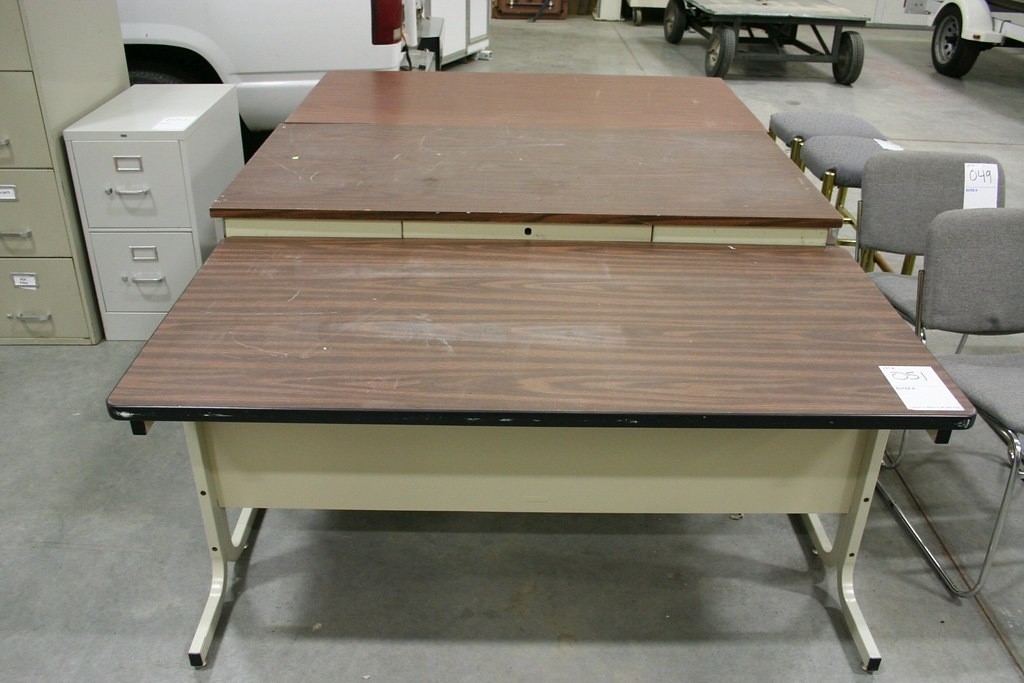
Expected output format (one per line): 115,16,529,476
109,70,978,675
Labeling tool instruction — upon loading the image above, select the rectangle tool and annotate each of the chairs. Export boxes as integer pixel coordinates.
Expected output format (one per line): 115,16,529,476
859,153,1024,607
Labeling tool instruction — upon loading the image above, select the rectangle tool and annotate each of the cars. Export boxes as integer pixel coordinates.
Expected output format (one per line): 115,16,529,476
919,0,1023,79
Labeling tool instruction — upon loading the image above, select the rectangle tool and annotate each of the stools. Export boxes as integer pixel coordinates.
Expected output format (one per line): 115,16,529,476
768,111,906,246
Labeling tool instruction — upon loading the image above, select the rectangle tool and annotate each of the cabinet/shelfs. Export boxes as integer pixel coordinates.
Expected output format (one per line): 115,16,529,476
0,0,244,347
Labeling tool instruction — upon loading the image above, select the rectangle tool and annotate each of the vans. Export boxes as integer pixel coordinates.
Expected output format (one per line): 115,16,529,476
118,1,407,156
